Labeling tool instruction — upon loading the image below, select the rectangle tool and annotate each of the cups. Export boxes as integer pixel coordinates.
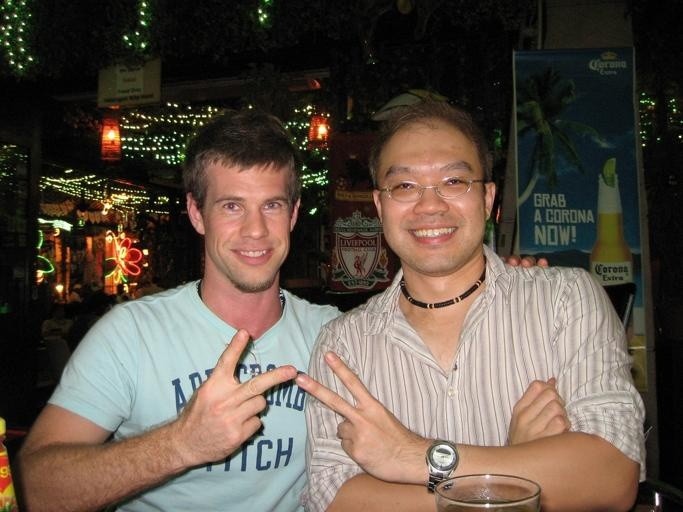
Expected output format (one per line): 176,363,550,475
433,473,543,511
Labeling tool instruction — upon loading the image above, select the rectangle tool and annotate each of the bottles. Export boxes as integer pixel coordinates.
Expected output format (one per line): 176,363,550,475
589,172,635,333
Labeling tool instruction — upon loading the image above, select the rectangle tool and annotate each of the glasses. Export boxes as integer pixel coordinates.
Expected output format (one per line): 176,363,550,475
377,176,484,203
